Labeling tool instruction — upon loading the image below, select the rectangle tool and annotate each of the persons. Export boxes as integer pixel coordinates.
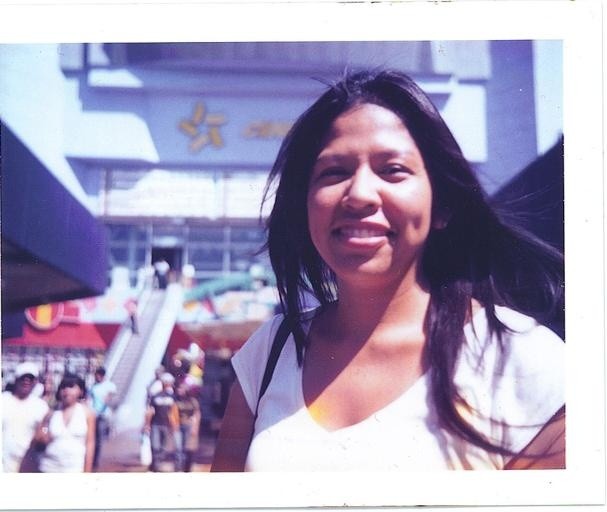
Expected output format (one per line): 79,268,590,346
211,67,566,469
0,349,207,472
155,256,169,289
123,292,142,336
181,260,196,289
